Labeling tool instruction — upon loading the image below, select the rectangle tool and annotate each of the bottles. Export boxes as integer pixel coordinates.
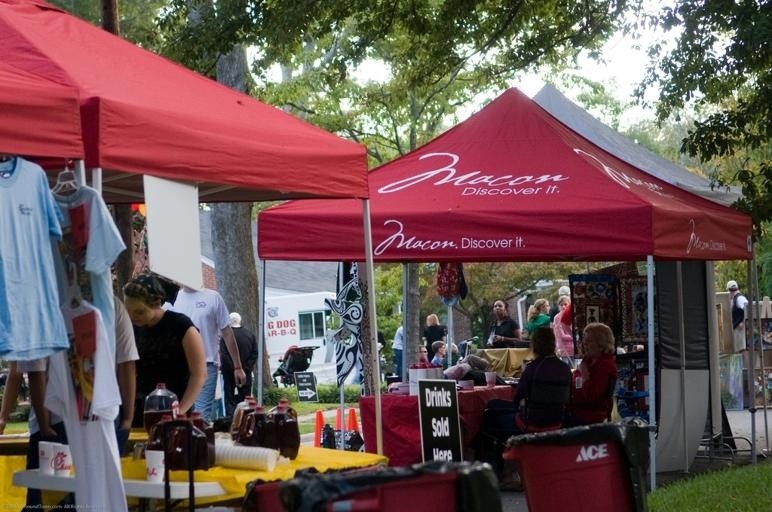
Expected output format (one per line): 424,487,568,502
231,395,270,446
142,382,213,471
272,398,300,460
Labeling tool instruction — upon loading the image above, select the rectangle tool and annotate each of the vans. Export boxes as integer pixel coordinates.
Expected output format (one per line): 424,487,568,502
265,291,356,392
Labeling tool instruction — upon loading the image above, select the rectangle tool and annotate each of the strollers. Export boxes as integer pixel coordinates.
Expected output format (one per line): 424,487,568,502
272,346,321,388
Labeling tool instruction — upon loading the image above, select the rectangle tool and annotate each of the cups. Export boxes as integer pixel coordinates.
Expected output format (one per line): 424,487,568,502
145,450,167,483
38,441,71,479
215,443,279,473
485,371,497,388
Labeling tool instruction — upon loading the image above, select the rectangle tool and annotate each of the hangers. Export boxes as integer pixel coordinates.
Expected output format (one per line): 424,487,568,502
51,157,81,194
1,152,18,175
66,262,84,308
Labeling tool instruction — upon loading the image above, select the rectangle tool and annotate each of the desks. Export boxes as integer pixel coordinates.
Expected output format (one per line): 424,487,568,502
0,427,390,511
359,384,517,468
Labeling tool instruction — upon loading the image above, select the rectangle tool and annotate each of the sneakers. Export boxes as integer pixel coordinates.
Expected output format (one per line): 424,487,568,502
502,481,523,491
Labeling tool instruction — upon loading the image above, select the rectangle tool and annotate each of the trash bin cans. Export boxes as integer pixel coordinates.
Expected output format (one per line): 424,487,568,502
241,461,501,512
502,417,657,512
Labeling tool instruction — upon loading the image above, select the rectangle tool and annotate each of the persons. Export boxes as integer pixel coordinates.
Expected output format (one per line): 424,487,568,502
494,327,571,491
218,312,258,414
120,279,207,429
569,322,616,427
172,283,247,429
392,285,576,385
24,293,141,512
726,279,749,369
0,361,29,435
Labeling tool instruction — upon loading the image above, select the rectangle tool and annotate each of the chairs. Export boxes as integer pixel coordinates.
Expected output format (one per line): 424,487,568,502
497,356,573,491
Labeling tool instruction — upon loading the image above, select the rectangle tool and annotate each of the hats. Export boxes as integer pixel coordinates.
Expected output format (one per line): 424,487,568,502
559,286,571,295
727,280,737,290
230,312,241,328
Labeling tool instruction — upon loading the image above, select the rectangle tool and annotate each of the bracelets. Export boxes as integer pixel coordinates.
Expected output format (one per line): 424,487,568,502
235,366,243,370
